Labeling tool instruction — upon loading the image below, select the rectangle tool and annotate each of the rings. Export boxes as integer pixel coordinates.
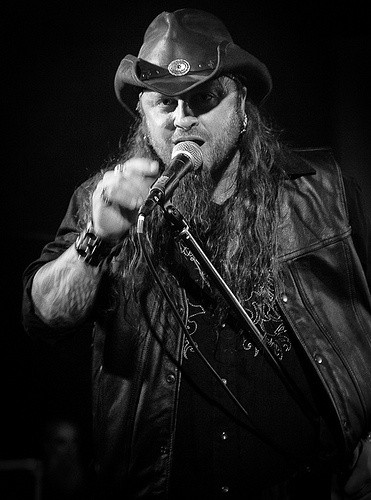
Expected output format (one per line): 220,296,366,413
119,162,124,174
100,185,113,206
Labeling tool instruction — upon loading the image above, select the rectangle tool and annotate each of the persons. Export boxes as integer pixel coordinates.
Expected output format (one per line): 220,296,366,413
22,8,371,500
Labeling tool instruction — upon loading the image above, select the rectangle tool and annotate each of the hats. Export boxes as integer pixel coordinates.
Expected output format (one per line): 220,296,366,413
114,7,272,119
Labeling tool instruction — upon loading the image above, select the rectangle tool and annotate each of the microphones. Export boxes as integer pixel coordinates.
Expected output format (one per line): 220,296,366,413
137,141,203,219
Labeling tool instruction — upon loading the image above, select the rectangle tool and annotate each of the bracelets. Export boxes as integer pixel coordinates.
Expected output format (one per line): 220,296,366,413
74,217,130,266
